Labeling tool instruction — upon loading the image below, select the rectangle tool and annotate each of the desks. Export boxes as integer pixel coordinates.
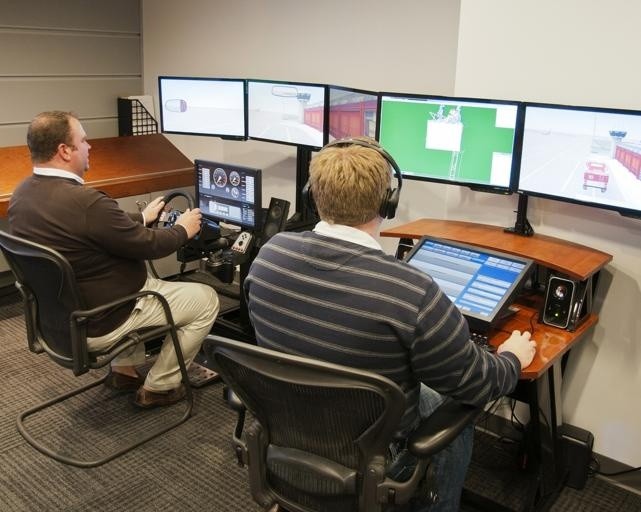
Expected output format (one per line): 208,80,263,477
0,133,195,218
381,220,614,512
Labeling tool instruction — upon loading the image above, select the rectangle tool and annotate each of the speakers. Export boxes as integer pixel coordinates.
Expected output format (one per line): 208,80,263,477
261,197,290,248
542,276,576,329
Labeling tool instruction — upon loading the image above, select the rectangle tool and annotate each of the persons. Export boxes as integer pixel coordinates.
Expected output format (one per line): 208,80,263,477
241,136,538,512
8,111,221,409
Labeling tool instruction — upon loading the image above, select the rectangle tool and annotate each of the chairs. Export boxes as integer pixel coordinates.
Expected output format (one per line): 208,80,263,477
0,231,194,467
203,336,490,512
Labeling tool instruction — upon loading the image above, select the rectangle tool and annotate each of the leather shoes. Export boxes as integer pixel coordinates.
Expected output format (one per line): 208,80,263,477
104,367,186,408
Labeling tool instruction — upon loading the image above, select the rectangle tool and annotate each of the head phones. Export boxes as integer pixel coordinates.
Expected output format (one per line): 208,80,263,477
301,140,402,219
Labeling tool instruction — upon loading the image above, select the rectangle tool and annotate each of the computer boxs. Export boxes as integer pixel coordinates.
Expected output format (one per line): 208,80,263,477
517,416,595,490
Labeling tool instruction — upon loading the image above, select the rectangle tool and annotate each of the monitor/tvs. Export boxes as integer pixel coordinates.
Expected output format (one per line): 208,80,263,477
512,102,641,215
378,92,522,192
194,159,261,232
158,76,247,139
327,84,379,143
247,79,326,150
403,235,536,325
